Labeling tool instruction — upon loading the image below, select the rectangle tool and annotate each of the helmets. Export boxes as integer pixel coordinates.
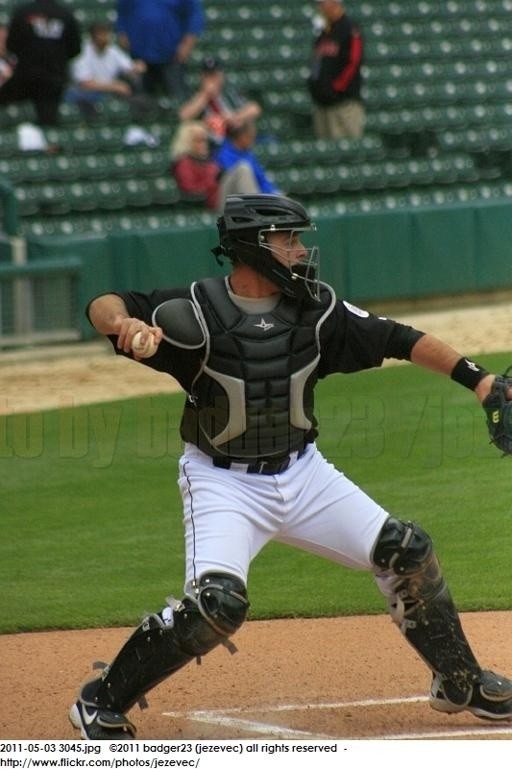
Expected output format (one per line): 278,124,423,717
216,187,319,243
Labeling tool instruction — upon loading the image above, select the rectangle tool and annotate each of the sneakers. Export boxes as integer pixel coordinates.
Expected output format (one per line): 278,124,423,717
424,668,512,725
66,677,138,740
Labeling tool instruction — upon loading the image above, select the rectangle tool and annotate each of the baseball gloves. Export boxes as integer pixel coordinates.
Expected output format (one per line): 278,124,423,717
482,374,512,456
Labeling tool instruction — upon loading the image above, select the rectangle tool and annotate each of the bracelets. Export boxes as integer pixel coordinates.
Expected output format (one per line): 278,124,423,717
451,356,492,392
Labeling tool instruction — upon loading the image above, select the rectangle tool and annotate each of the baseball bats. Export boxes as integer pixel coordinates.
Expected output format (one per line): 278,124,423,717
131,331,158,360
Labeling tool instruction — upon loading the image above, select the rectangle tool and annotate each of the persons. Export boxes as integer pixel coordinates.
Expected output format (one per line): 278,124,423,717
203,118,285,199
63,189,512,739
168,122,263,212
177,53,263,146
307,0,368,142
0,1,206,160
